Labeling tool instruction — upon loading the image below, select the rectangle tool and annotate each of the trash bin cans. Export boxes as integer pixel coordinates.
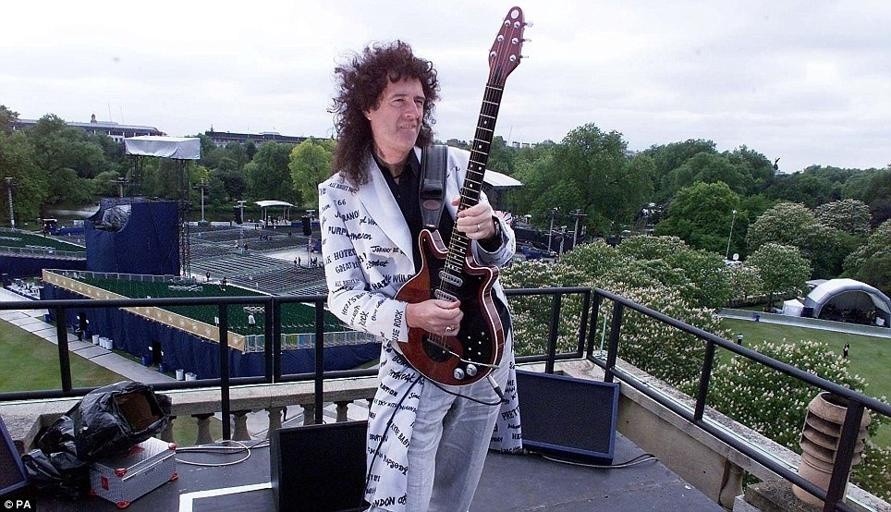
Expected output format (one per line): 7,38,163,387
44,314,52,323
83,329,90,338
176,369,197,382
92,334,113,350
72,323,79,333
159,362,168,373
141,354,149,366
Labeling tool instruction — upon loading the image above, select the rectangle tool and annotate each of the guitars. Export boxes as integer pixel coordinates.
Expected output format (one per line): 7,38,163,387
393,6,532,387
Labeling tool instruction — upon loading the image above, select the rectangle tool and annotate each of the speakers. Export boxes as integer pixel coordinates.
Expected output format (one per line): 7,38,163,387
516,369,622,463
269,420,373,512
1,418,27,495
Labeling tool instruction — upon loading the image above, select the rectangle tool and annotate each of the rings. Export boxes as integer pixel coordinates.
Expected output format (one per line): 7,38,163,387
476,223,481,231
446,325,452,332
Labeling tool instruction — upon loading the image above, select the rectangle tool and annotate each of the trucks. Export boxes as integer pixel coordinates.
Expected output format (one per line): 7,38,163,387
43,217,86,236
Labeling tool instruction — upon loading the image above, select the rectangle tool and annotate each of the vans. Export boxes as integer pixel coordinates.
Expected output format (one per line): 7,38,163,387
620,229,632,239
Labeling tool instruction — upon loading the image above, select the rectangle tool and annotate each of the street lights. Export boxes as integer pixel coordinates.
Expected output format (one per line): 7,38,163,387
724,207,740,260
4,175,16,229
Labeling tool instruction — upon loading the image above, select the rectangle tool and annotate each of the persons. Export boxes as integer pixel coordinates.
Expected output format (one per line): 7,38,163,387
843,341,851,358
294,256,298,267
219,284,222,291
206,271,211,282
223,276,227,287
72,320,86,342
248,312,256,327
288,228,292,238
737,331,744,345
752,312,761,326
214,313,220,326
49,223,53,235
235,243,240,249
43,223,48,238
298,256,301,267
316,39,526,511
241,242,249,251
